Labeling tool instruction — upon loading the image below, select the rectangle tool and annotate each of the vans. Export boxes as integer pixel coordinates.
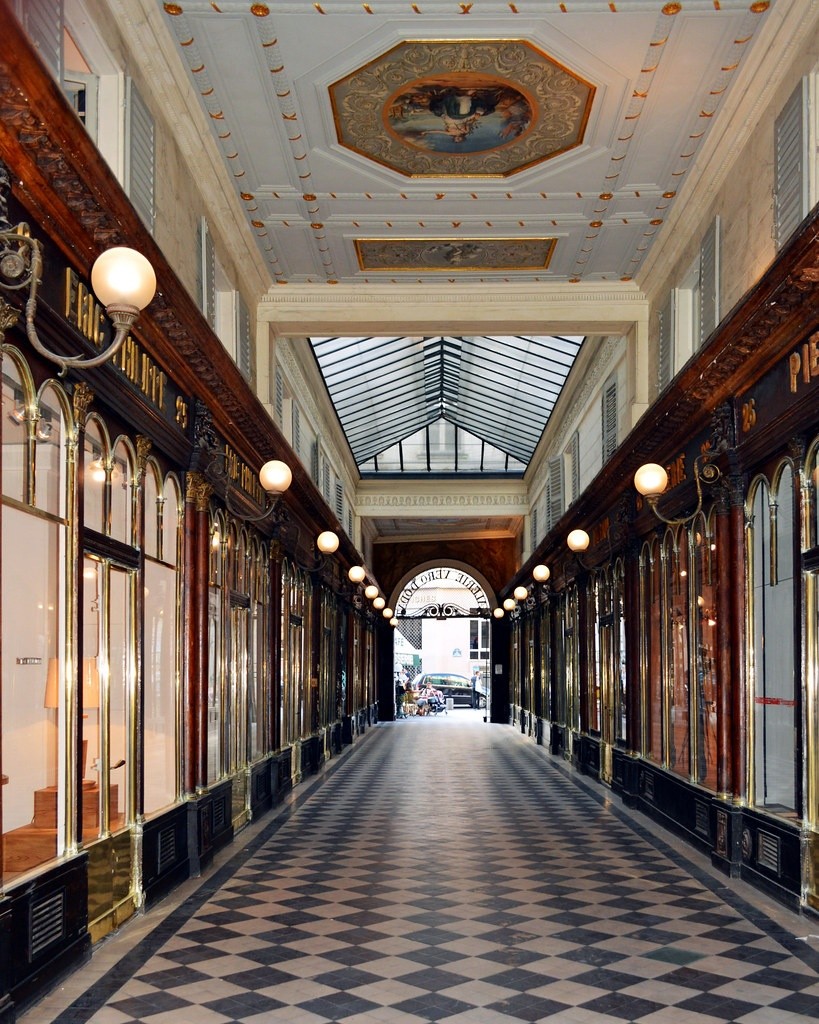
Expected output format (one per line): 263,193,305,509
411,673,487,707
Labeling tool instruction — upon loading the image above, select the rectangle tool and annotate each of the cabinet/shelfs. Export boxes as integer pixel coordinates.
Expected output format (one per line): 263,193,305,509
2,783,125,872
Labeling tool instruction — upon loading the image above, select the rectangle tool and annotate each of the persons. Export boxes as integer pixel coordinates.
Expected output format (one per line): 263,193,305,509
396,668,444,719
471,671,480,709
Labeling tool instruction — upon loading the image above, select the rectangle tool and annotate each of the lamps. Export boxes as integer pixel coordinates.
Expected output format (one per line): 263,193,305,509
205,452,293,522
0,222,156,378
566,523,621,571
493,586,537,623
634,455,719,526
332,566,398,629
291,525,339,573
44,657,99,791
533,565,568,595
